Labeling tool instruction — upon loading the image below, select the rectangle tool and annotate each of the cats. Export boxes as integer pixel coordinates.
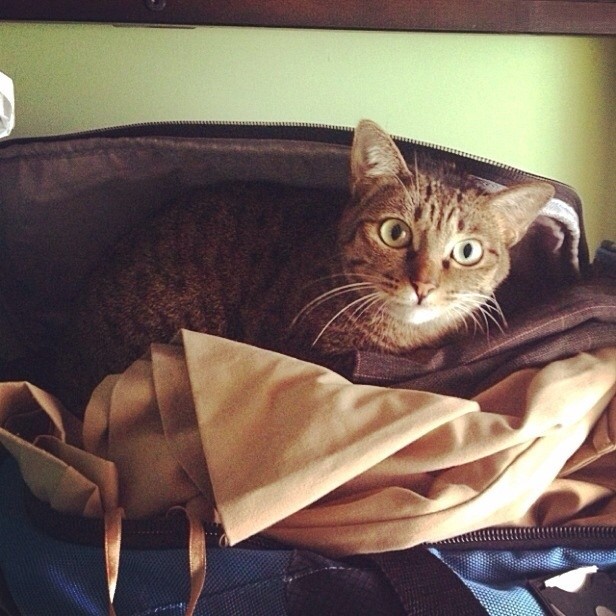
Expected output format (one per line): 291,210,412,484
0,119,558,422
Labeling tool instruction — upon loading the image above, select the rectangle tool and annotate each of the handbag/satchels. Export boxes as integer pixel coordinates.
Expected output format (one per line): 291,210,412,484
2,121,615,615
